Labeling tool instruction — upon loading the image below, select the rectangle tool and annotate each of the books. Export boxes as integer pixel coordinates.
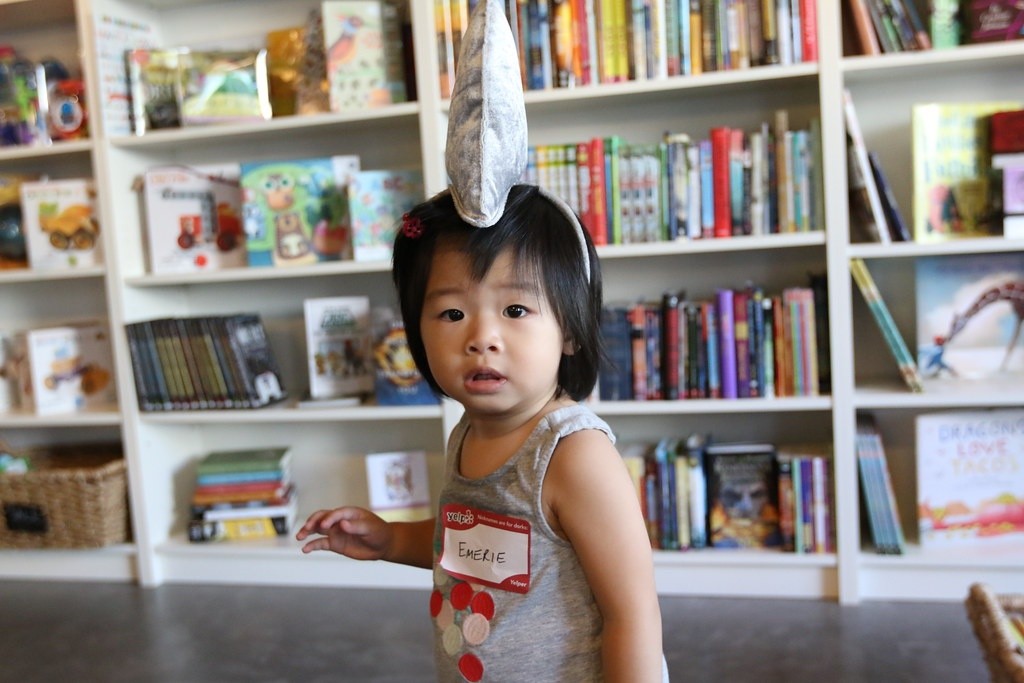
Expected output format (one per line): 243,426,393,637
850,254,1024,391
520,111,823,245
188,447,301,542
322,0,409,111
1,325,114,416
125,313,288,411
857,407,1024,554
0,171,107,271
435,0,819,98
843,0,1024,54
582,272,830,402
143,154,424,275
621,430,835,555
296,297,441,406
844,88,1024,244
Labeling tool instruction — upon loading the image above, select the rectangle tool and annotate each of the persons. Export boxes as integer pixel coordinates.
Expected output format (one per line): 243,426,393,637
294,184,669,683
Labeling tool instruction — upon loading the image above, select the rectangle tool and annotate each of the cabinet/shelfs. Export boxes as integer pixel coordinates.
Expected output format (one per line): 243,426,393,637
1,2,1024,597
